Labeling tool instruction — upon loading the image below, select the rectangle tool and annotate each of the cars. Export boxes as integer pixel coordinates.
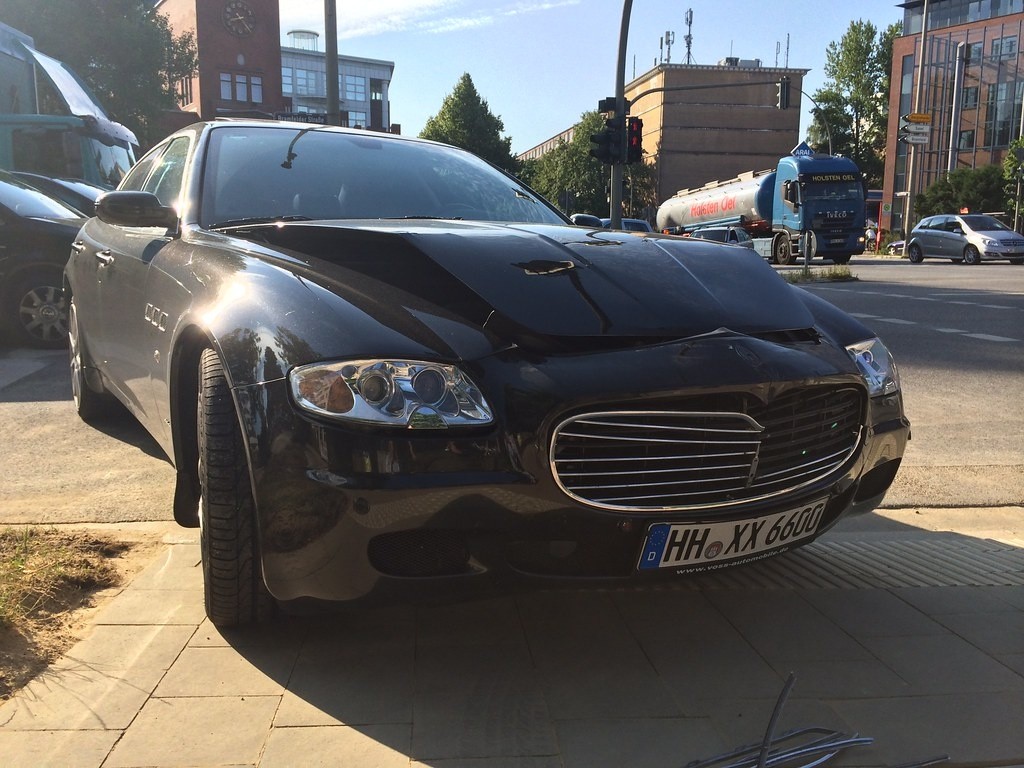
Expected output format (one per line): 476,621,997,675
908,215,1024,265
61,116,914,626
0,169,90,349
688,227,755,249
600,218,652,232
887,240,904,255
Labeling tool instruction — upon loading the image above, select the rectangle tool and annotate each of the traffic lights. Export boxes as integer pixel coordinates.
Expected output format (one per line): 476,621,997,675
605,118,625,163
589,133,608,163
1007,178,1017,200
628,117,643,162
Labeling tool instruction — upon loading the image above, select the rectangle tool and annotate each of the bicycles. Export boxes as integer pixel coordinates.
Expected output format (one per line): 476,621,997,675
864,240,876,255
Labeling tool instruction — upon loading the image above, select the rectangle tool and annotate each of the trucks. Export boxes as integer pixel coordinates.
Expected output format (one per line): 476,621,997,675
660,154,868,264
0,20,139,191
